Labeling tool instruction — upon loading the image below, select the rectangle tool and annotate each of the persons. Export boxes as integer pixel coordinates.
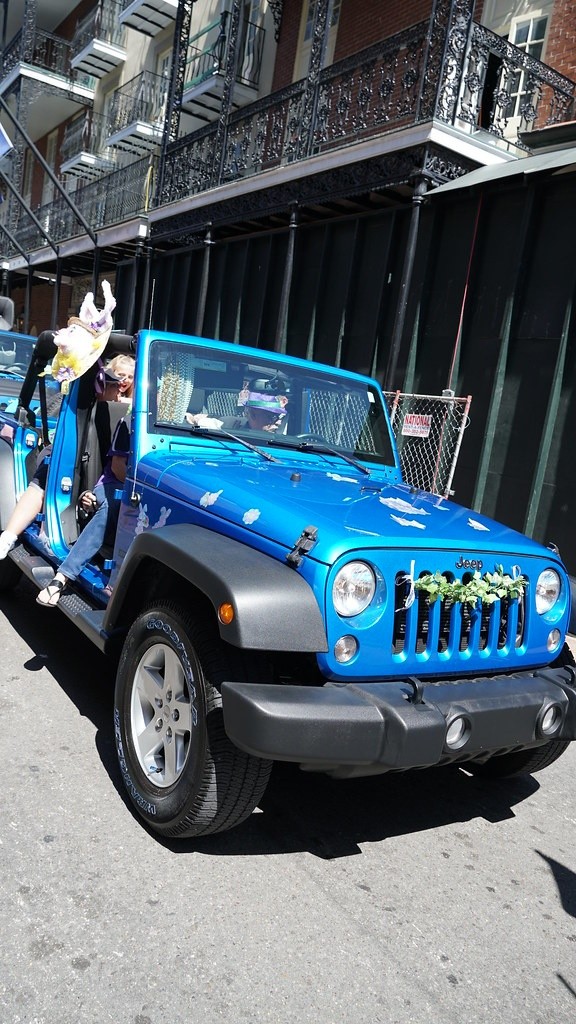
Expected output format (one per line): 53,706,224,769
0,352,289,608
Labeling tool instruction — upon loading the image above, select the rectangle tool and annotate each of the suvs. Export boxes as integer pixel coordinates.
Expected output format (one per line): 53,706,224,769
0,328,576,854
0,329,63,441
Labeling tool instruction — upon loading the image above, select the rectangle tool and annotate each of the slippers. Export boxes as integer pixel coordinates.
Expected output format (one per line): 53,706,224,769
36,578,65,609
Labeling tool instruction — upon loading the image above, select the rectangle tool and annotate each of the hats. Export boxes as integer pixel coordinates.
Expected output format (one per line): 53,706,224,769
241,391,288,416
37,278,117,394
99,368,126,386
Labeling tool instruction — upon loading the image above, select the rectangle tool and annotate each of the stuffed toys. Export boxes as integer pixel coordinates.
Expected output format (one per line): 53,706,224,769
38,280,118,397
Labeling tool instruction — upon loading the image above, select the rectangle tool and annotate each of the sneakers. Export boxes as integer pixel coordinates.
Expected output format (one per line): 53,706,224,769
0,530,18,560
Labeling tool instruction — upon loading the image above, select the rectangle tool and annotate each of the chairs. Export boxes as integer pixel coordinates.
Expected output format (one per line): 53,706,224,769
84,389,210,504
